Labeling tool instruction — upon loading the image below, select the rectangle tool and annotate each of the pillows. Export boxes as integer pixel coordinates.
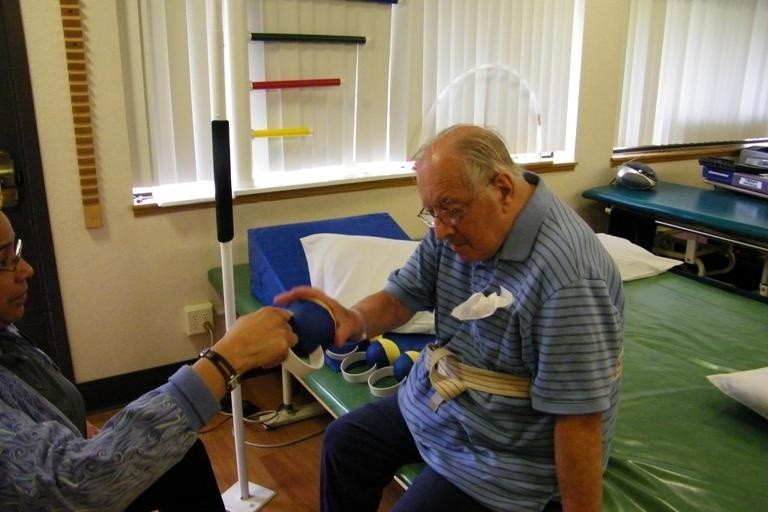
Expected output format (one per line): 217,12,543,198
249,213,412,310
298,231,435,334
593,231,684,285
706,366,768,419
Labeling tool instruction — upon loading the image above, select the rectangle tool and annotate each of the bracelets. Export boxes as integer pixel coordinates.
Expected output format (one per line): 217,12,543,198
197,349,243,393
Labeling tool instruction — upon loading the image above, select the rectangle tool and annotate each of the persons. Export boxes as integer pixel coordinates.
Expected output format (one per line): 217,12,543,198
273,122,626,512
0,207,301,510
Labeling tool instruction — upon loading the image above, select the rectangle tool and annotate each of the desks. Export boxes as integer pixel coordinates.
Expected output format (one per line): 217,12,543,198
583,179,767,297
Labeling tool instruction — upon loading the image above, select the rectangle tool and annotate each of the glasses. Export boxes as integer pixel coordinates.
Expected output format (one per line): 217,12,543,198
417,177,495,227
0,238,23,271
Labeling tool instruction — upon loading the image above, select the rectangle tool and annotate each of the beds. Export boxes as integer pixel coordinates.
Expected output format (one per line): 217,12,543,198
208,262,768,512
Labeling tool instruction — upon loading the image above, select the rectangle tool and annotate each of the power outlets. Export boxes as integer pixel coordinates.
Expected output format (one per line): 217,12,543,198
182,301,214,338
